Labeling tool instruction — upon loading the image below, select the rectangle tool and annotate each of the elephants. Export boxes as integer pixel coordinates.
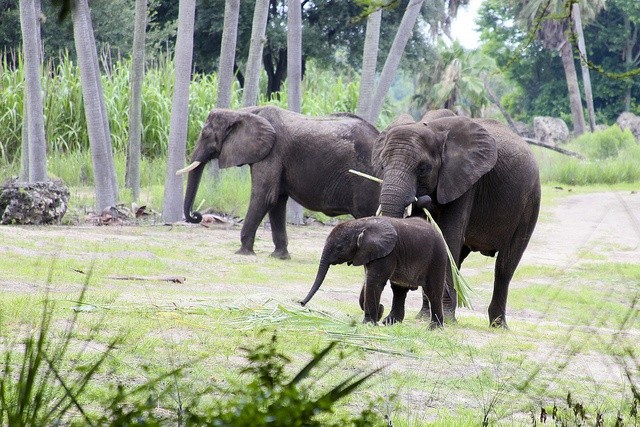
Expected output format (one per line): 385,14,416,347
175,104,384,260
370,107,541,331
299,215,449,331
